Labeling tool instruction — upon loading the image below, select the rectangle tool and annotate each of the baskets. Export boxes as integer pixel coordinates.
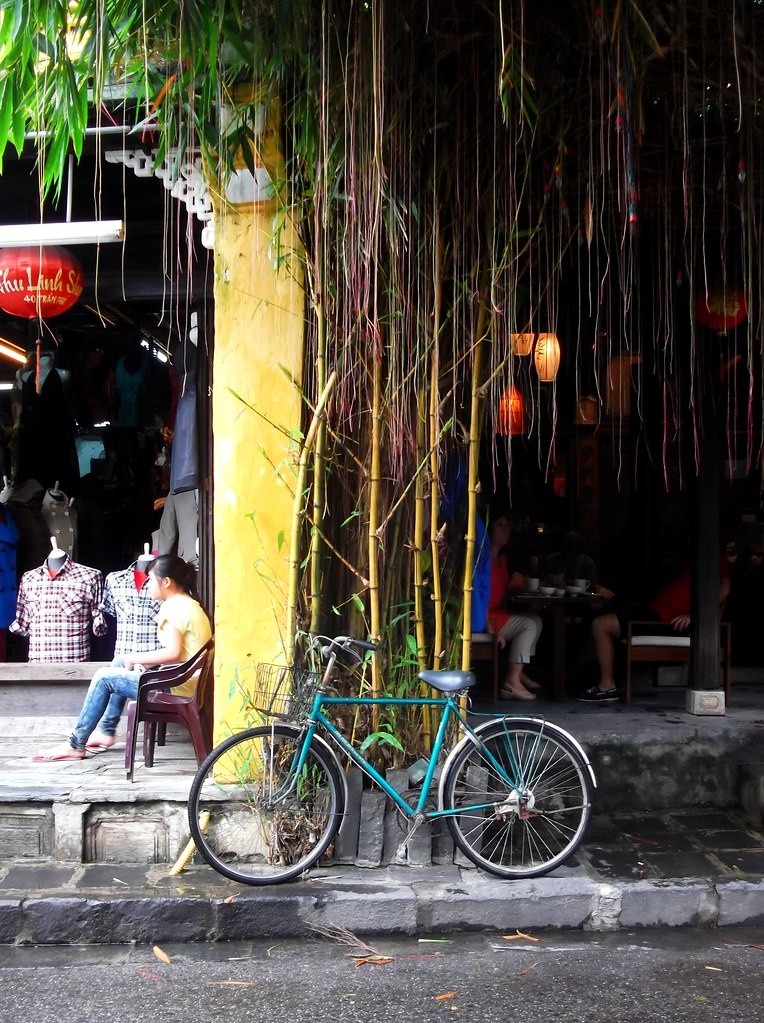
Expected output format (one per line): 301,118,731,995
255,663,324,722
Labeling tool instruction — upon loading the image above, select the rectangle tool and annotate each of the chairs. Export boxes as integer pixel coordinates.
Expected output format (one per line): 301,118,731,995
468,619,512,703
126,633,215,784
627,597,731,706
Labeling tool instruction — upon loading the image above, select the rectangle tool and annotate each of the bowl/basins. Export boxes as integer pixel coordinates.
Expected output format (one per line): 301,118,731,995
555,589,566,598
568,587,581,597
539,587,555,597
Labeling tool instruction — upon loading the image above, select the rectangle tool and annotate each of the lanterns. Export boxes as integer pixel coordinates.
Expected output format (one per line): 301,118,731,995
692,272,750,336
0,243,85,320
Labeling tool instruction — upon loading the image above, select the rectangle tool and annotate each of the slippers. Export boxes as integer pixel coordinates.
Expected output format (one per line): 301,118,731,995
32,753,82,761
85,745,107,754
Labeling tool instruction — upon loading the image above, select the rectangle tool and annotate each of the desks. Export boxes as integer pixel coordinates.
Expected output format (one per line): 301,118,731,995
515,592,606,701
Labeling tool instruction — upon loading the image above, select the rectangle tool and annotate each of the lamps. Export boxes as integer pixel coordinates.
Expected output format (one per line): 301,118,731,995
495,382,525,434
535,333,560,382
510,334,533,355
0,155,124,248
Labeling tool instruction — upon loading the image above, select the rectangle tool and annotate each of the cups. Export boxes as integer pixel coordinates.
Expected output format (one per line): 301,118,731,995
527,578,539,592
575,579,590,594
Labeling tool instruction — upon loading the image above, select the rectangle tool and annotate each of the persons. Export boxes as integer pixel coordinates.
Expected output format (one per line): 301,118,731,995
484,511,543,702
1,479,169,665
571,555,732,701
34,554,212,761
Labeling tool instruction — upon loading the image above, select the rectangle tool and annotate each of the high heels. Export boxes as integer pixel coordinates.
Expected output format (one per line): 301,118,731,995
523,678,541,689
500,682,536,700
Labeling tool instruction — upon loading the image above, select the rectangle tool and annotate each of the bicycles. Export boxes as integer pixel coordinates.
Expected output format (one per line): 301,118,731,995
187,634,597,887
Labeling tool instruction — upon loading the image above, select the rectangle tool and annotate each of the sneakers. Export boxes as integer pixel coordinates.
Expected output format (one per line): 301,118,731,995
608,687,620,701
576,686,609,701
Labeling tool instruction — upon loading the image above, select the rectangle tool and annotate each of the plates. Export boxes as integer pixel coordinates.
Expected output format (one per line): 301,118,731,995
521,593,535,595
